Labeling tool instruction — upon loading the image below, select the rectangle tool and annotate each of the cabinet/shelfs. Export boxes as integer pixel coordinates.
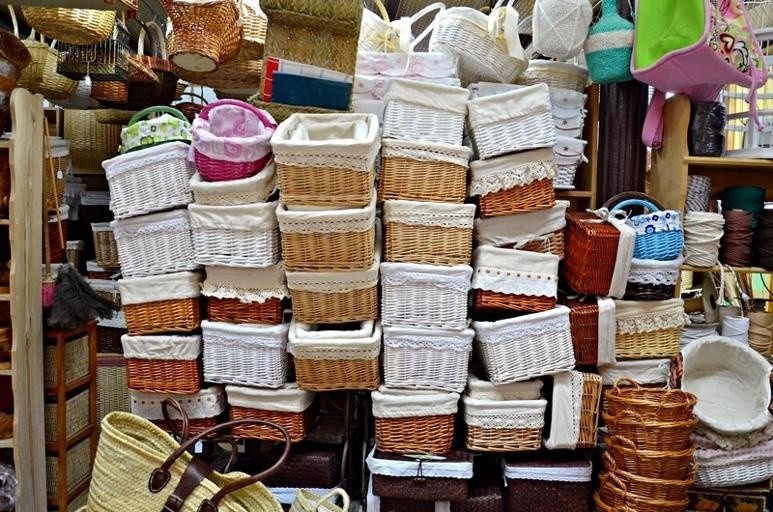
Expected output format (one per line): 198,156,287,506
0,86,98,512
642,92,773,335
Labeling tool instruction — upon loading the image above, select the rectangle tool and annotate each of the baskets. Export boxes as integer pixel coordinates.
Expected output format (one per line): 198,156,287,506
119,332,203,394
517,60,589,95
158,1,269,92
275,187,378,274
117,269,201,338
258,0,530,85
189,200,279,269
380,75,773,512
268,109,381,212
190,162,275,206
190,100,277,181
285,216,383,324
364,75,773,512
198,319,290,390
101,141,195,222
202,262,287,325
286,315,384,393
224,383,314,445
108,209,202,283
0,0,190,212
2,221,134,509
0,0,190,217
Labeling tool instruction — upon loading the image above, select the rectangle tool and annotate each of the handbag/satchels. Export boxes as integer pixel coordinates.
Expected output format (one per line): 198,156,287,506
84,397,291,511
628,0,773,100
289,486,353,512
